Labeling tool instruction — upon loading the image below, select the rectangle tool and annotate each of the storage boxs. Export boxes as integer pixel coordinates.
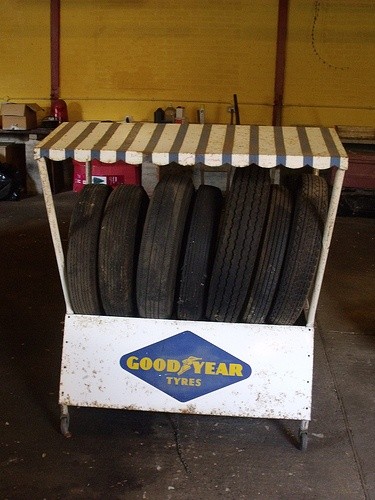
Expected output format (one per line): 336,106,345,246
1,103,44,130
